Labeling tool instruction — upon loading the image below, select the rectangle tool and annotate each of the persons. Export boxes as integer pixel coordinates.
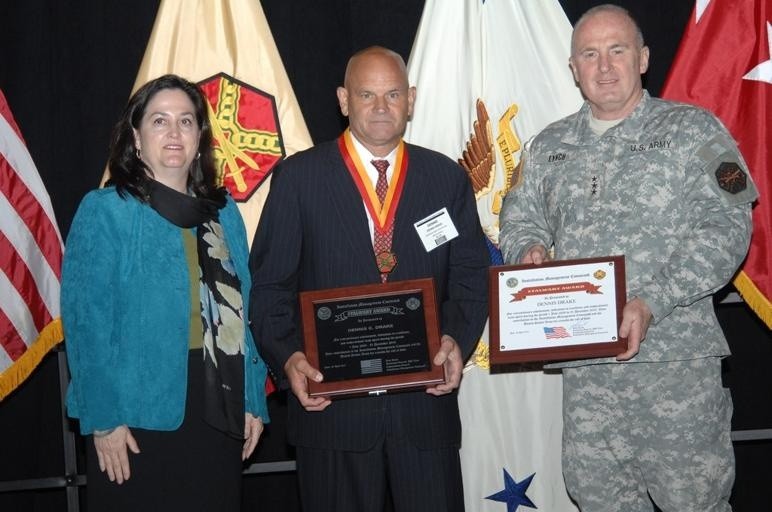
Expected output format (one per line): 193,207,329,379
499,6,762,512
62,74,272,511
248,45,492,512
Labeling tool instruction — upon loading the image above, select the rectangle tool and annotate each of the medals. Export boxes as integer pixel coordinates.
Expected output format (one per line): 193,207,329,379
376,251,397,273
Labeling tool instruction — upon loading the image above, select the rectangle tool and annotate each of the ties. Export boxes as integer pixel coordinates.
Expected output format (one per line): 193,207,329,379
370,160,395,283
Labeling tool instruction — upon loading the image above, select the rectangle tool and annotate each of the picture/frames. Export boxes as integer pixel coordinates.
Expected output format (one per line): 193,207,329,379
297,278,445,399
486,254,626,367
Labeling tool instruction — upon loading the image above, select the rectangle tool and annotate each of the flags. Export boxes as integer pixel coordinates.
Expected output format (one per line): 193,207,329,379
656,1,772,331
0,88,64,405
94,1,318,396
405,1,587,511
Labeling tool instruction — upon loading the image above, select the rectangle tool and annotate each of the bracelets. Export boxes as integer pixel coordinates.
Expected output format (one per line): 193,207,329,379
92,427,117,437
